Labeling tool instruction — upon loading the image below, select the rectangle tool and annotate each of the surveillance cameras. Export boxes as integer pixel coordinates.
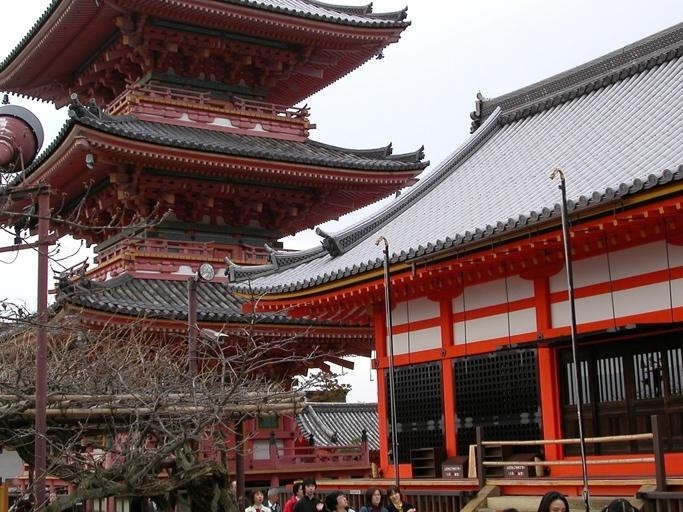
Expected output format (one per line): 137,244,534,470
199,327,229,345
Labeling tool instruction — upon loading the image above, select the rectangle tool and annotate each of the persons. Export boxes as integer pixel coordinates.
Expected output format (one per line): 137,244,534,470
216,477,416,511
45,490,58,506
601,496,639,512
537,491,569,512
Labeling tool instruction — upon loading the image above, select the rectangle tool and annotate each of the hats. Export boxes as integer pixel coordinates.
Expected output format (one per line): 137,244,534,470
603,498,637,512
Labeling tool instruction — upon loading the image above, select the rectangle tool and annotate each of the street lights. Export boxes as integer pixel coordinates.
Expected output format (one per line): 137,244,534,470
371,232,402,495
548,167,593,512
186,261,216,381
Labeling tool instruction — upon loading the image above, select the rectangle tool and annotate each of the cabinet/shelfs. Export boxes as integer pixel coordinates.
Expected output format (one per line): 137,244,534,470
474,445,512,478
410,447,442,479
